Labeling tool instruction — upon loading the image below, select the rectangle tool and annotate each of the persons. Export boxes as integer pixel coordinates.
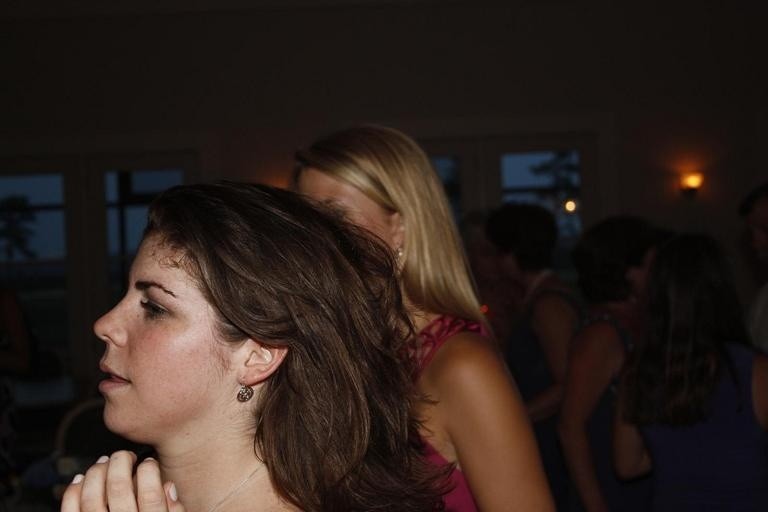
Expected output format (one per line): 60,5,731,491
461,184,768,512
59,180,459,512
0,289,50,381
292,121,557,512
